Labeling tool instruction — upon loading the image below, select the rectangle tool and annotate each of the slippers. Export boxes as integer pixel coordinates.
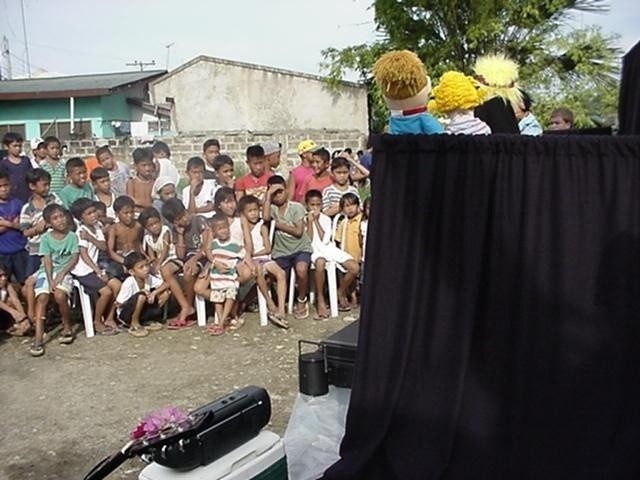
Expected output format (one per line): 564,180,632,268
58,329,75,344
29,339,44,356
92,301,351,335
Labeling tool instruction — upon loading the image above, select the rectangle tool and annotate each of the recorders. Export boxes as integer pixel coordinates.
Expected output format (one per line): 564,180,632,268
137,385,271,470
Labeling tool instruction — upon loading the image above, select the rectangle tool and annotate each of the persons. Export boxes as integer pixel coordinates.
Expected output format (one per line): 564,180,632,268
516,89,543,135
551,108,573,130
0,134,370,355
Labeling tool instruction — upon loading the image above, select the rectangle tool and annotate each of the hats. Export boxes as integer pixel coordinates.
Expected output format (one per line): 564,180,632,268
297,139,324,156
30,136,45,150
154,177,175,192
95,138,109,148
260,141,282,156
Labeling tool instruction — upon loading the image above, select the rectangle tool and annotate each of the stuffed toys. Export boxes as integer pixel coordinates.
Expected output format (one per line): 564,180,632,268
375,49,443,135
474,49,515,129
430,70,491,135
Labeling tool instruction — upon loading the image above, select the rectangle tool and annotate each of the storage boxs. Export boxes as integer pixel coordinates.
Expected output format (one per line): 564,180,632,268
138,430,288,480
